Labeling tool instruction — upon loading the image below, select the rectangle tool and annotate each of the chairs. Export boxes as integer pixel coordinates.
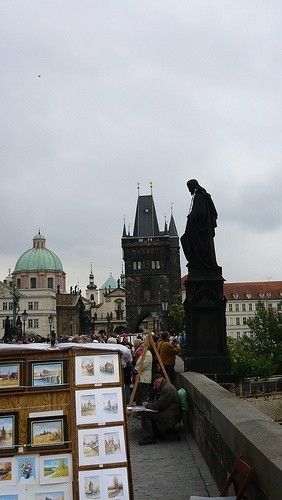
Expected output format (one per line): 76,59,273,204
158,388,188,441
190,455,253,500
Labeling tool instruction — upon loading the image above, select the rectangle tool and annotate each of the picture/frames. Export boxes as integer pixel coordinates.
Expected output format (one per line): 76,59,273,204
0,353,133,500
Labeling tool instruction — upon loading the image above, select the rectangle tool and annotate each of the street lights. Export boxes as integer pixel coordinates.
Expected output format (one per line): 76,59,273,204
160,296,170,332
155,311,160,335
21,309,28,344
69,317,74,336
151,306,158,334
48,314,54,341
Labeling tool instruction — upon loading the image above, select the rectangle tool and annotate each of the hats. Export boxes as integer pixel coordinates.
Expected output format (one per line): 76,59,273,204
162,331,171,339
134,339,145,353
150,374,163,384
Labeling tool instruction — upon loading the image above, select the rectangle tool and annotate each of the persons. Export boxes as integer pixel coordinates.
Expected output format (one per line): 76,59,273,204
5,316,10,333
161,332,180,384
136,375,181,445
135,342,153,405
17,316,22,326
120,341,132,405
150,331,186,345
0,333,160,344
180,179,220,267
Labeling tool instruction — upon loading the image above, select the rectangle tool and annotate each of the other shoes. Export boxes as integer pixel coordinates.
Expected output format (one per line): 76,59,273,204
139,436,158,446
